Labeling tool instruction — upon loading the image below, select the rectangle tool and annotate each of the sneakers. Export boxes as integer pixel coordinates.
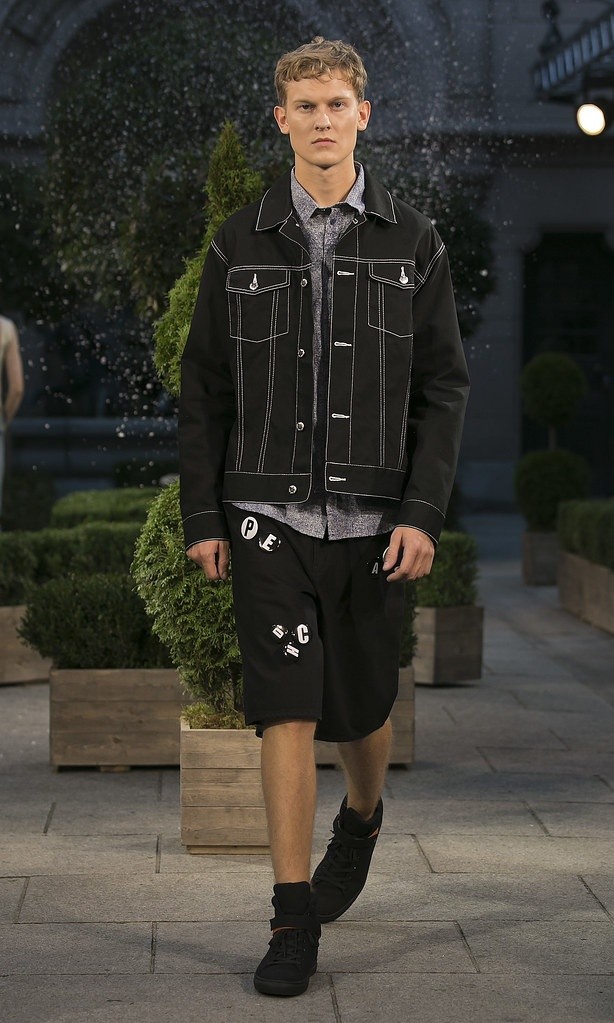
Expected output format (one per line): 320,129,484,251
309,792,383,922
254,914,322,996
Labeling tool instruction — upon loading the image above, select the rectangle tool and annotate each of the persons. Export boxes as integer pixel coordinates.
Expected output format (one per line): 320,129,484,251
175,33,471,996
0,315,25,434
